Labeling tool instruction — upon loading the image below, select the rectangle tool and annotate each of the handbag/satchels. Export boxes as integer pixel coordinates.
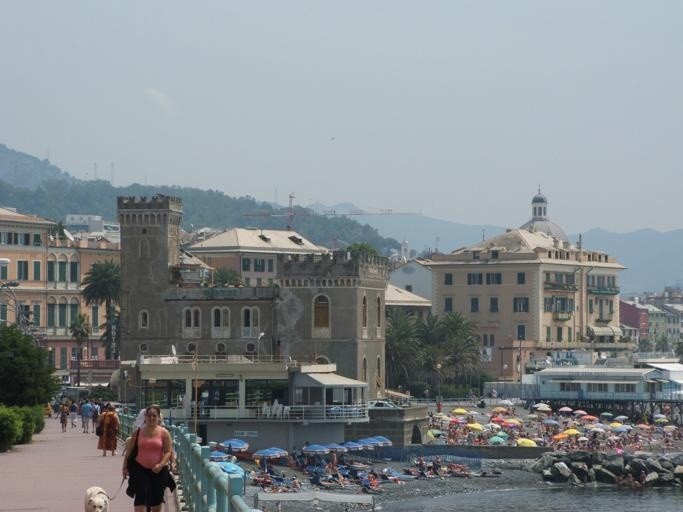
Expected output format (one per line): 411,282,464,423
95,425,103,436
127,427,139,462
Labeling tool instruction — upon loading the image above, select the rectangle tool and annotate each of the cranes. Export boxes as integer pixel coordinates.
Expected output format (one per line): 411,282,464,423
241,193,421,232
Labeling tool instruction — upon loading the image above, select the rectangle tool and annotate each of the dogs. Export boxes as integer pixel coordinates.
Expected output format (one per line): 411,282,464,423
121,437,132,456
85,486,109,512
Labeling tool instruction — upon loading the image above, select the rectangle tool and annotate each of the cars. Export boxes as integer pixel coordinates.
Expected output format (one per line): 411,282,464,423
322,399,404,418
42,396,79,417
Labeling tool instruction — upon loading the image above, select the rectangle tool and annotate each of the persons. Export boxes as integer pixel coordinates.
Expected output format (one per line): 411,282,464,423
121,406,172,511
51,390,166,458
252,450,470,494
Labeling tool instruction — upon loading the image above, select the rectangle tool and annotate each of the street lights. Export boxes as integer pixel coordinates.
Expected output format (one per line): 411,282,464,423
502,364,510,400
258,332,266,364
1,279,20,287
75,346,86,388
48,346,54,375
436,364,443,406
